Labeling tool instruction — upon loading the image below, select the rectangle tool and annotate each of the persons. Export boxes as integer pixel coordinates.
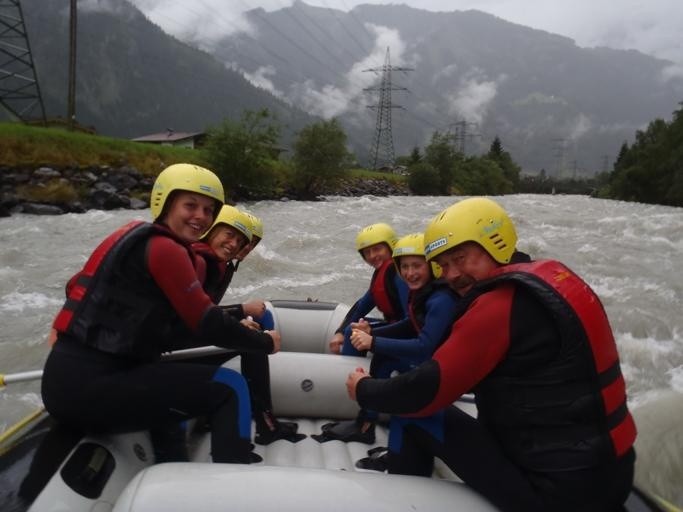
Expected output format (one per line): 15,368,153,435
210,212,298,433
168,204,308,444
41,162,281,465
344,196,637,512
323,232,458,470
329,223,412,357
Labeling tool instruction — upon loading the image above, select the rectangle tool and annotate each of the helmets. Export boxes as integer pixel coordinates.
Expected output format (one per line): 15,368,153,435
151,164,264,244
355,197,518,279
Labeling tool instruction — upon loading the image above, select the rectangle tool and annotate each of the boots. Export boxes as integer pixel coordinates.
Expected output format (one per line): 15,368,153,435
326,409,378,444
355,450,388,473
254,409,298,445
